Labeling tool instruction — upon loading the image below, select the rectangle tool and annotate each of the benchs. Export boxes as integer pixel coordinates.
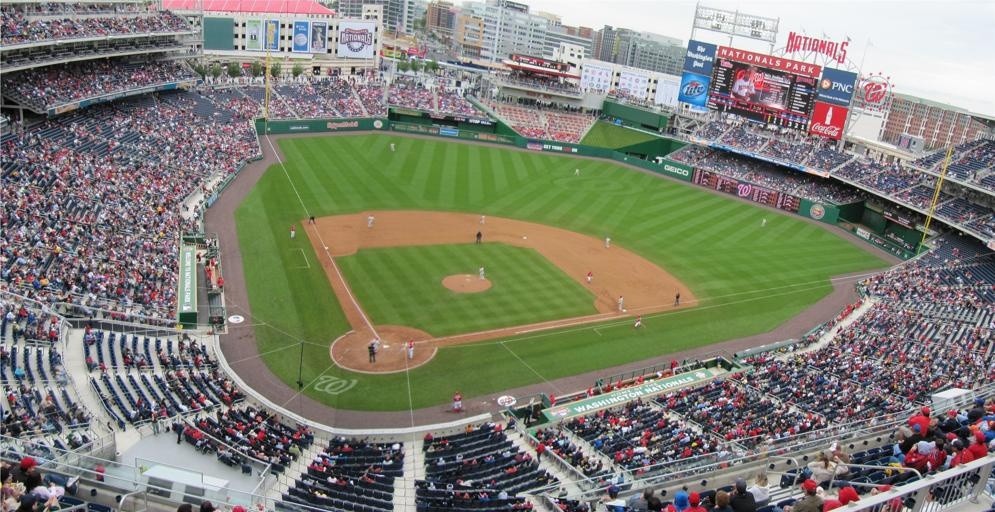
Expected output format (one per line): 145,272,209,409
0,1,995,512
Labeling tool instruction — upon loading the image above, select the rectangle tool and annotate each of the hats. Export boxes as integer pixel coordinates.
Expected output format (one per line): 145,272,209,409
688,491,700,505
672,490,689,511
911,398,986,447
822,498,843,511
736,477,746,489
20,456,37,469
800,478,817,491
608,484,620,496
839,486,860,504
200,500,216,512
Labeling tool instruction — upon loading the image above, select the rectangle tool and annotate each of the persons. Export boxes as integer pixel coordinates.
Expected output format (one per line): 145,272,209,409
840,115,994,512
605,236,611,248
760,216,767,227
264,295,854,512
264,64,883,211
479,265,485,281
479,214,486,225
290,223,296,240
585,271,593,283
476,231,482,245
674,292,680,307
0,0,264,512
308,215,315,225
367,214,375,227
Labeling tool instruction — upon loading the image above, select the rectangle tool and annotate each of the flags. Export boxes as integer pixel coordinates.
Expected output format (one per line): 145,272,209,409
397,17,403,32
